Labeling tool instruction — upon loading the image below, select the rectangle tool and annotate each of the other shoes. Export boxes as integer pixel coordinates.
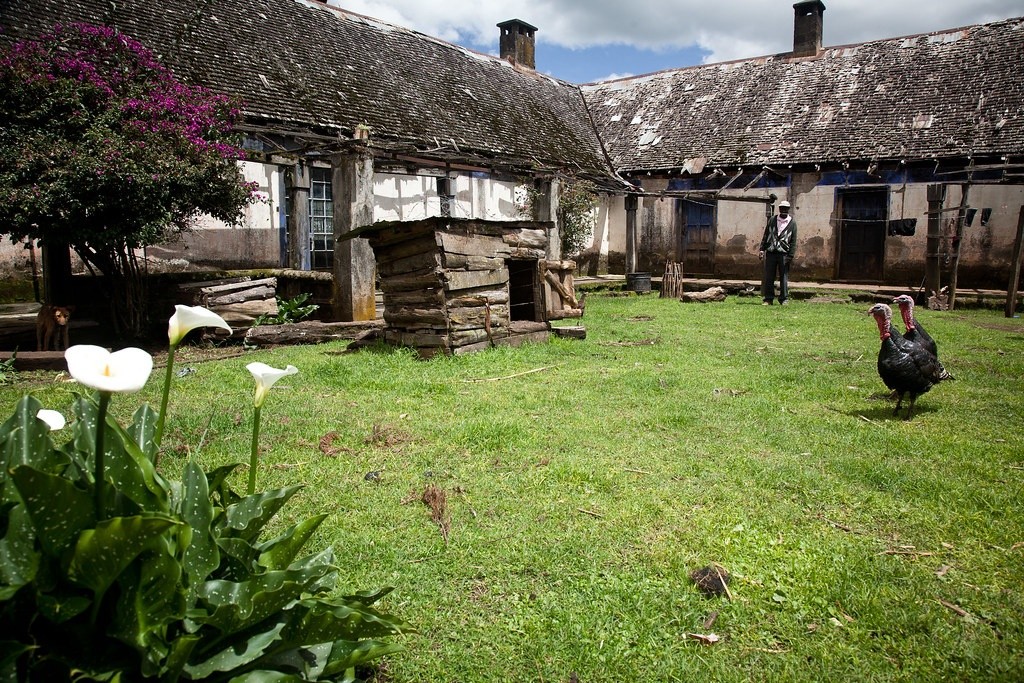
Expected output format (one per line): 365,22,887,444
762,301,770,305
782,300,789,306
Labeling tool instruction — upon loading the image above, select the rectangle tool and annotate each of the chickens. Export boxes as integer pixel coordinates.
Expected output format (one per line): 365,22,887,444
868,294,955,421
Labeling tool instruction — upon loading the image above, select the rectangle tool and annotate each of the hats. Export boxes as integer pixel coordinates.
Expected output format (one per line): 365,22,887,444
778,201,790,207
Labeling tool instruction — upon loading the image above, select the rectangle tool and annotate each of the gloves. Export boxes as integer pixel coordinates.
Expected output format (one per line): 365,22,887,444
759,251,764,260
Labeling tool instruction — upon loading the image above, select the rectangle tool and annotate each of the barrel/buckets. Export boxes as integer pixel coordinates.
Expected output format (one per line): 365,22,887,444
626,273,651,292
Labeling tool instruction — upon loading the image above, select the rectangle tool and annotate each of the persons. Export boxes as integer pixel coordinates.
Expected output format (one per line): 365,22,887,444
759,201,797,306
